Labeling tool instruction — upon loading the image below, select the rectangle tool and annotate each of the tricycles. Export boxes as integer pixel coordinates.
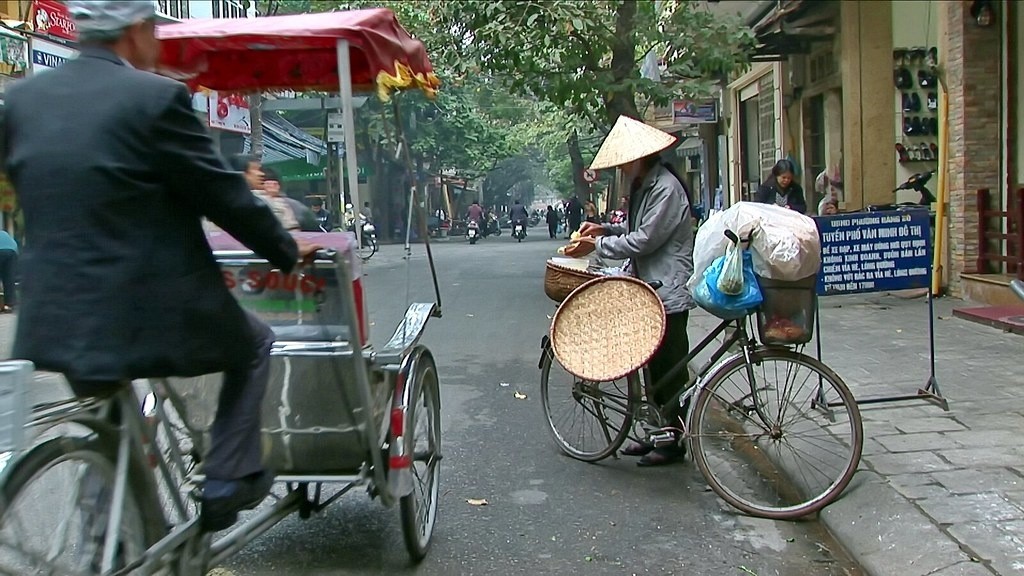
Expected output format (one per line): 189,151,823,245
0,7,444,576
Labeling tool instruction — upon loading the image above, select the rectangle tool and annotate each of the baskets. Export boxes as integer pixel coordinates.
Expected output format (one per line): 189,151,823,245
551,277,666,382
755,274,816,345
544,260,603,302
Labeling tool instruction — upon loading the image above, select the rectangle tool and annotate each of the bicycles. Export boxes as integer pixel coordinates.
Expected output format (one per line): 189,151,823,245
344,218,376,260
537,225,864,520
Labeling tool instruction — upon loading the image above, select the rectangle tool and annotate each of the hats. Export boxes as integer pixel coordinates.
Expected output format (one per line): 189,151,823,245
62,0,183,32
589,114,677,170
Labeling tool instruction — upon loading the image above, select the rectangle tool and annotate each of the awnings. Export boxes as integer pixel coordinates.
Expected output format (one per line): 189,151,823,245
242,110,336,165
718,0,840,62
446,179,475,191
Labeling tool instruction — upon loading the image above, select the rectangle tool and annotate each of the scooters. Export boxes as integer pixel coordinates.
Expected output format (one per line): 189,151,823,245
485,215,502,236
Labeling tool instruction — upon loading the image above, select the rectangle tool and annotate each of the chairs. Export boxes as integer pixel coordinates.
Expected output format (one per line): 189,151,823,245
202,231,440,363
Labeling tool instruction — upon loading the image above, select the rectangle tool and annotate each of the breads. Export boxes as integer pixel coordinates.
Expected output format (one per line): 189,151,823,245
764,327,803,341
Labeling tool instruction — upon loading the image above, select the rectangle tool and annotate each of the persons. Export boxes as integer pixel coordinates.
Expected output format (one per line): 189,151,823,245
563,115,697,466
0,0,326,576
0,154,373,314
436,197,628,240
819,197,837,214
754,159,807,214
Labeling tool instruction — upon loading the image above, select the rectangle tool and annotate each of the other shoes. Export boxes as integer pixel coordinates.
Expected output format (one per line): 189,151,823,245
893,46,938,66
927,92,937,112
902,93,921,112
917,67,938,88
904,116,921,136
893,67,913,89
189,467,273,531
921,117,938,137
895,142,938,162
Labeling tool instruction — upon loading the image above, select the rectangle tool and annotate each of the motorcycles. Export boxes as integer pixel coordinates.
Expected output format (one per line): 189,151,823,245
438,219,469,239
513,219,527,242
497,216,540,228
465,219,481,245
861,166,938,225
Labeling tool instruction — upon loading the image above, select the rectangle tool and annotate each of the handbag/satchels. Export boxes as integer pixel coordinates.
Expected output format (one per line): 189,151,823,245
692,235,764,321
